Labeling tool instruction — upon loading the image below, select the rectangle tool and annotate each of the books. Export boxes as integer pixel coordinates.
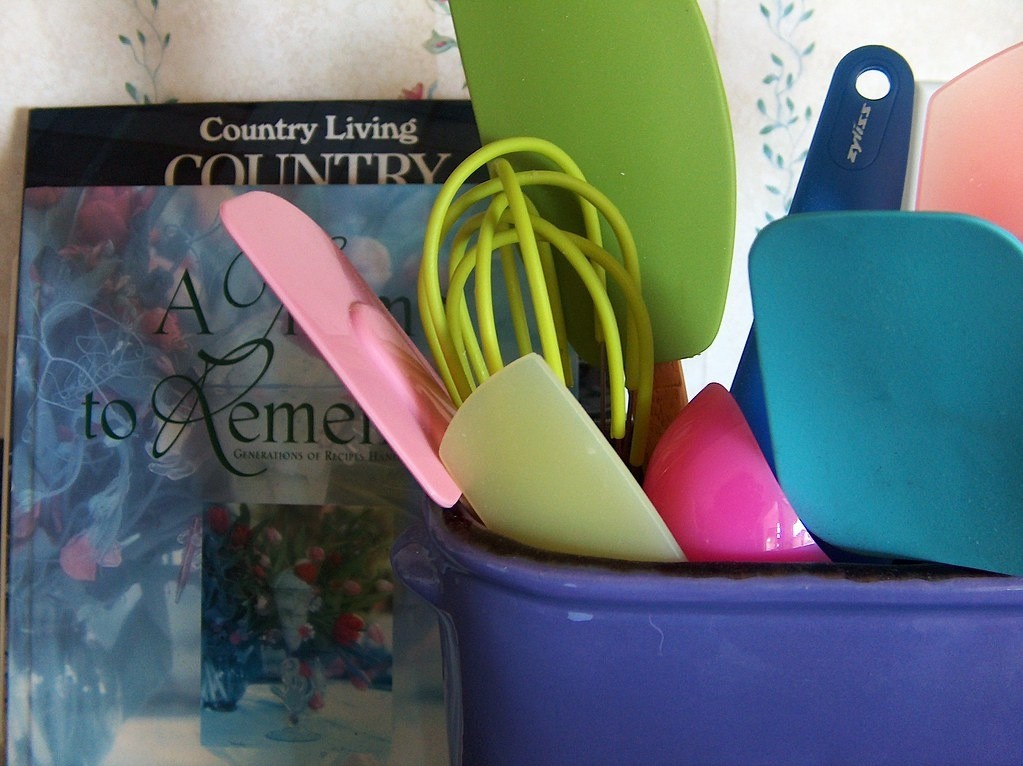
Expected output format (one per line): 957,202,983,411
6,97,549,766
